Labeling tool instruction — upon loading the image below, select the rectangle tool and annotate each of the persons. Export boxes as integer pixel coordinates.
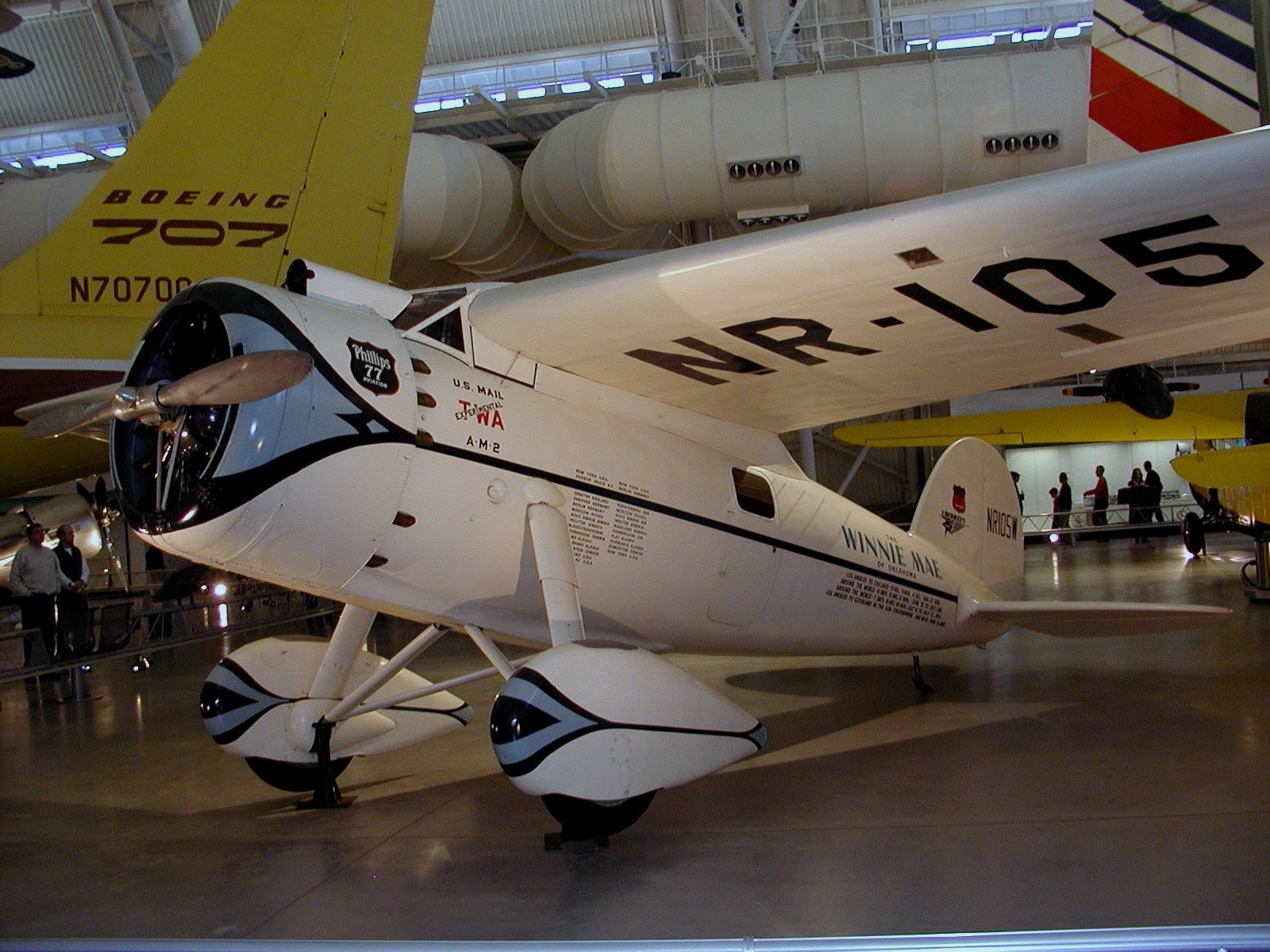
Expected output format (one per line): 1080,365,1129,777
1056,472,1080,549
1143,461,1164,522
52,523,92,675
10,524,66,705
1049,487,1059,529
1010,471,1025,514
1128,468,1150,544
1083,466,1111,542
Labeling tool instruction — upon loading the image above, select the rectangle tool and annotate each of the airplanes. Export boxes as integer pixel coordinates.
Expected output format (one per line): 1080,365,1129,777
0,123,1269,851
